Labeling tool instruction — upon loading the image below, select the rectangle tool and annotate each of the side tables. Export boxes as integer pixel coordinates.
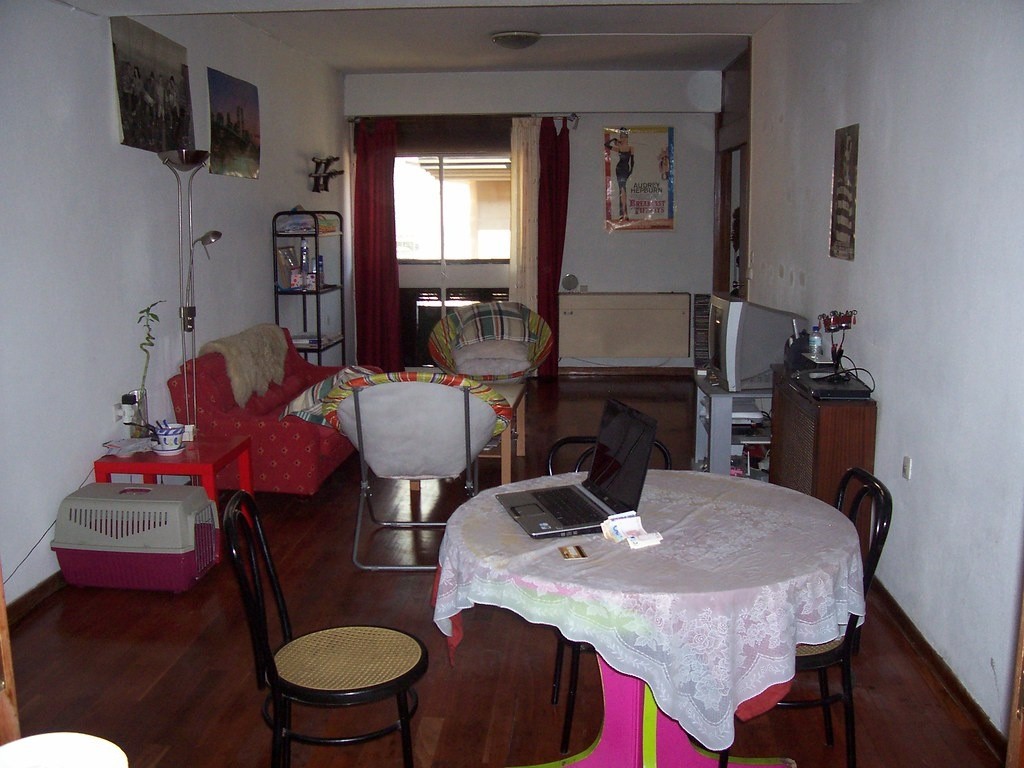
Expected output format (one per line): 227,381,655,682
94,433,254,565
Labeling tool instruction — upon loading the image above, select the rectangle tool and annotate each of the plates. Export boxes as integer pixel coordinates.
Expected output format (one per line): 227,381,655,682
153,443,185,456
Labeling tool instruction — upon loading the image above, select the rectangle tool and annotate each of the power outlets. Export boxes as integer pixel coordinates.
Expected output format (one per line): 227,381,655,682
903,456,912,480
113,405,126,421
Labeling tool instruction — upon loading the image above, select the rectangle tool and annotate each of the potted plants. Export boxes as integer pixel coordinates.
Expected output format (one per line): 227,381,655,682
129,300,167,435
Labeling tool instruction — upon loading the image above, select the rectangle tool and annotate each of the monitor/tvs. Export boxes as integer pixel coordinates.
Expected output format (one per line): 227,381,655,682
707,294,811,393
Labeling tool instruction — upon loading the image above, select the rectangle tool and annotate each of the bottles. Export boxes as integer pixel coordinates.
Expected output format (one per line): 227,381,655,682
300,238,308,273
318,255,323,284
809,326,822,354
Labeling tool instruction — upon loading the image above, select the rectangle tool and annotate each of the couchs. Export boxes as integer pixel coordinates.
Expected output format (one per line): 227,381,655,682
167,324,386,494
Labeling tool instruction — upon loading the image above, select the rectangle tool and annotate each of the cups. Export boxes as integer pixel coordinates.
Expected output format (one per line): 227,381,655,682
155,424,185,450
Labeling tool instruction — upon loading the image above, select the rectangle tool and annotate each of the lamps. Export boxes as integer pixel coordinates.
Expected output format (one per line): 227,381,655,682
159,149,222,435
493,32,540,51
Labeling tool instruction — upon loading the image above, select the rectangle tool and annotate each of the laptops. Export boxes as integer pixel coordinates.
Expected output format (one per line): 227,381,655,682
494,394,659,541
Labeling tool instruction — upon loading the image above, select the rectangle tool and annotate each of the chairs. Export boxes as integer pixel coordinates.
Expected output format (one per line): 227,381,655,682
428,302,553,443
689,466,893,768
322,370,513,572
0,564,133,768
224,489,426,768
550,436,675,754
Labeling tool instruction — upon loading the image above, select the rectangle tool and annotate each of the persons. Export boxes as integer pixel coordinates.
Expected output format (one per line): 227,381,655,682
605,131,634,221
128,67,180,131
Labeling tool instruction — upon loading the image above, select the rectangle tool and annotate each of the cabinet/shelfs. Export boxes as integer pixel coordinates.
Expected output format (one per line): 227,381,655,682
695,368,775,482
274,209,345,367
772,365,876,569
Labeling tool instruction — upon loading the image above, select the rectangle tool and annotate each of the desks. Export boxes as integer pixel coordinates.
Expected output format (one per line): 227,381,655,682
428,468,865,768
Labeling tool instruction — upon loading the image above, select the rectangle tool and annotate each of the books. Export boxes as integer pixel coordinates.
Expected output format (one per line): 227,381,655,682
293,339,324,349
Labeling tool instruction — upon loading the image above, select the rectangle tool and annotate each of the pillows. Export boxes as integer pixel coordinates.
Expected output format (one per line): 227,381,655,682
279,365,371,437
446,304,536,350
